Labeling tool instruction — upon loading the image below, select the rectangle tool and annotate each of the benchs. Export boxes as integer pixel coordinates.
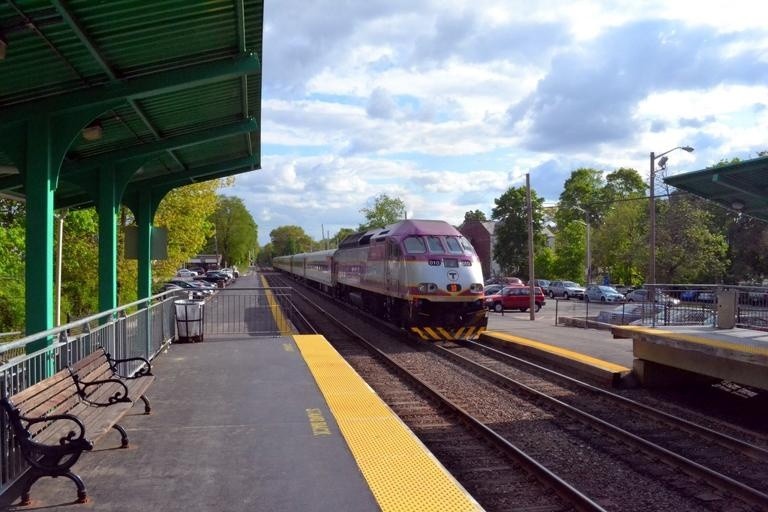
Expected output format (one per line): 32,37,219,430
66,346,155,449
0,366,132,506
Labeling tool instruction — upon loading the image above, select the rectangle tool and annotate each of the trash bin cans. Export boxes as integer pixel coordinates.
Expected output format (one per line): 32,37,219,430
174,300,204,342
718,292,736,330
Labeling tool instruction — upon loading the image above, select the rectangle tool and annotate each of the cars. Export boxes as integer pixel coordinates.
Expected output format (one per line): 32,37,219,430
158,265,239,302
484,276,768,329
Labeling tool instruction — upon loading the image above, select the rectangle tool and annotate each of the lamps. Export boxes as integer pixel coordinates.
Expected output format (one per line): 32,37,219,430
82,120,102,141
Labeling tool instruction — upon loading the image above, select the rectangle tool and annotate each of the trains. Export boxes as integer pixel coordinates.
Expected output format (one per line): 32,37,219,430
271,219,489,342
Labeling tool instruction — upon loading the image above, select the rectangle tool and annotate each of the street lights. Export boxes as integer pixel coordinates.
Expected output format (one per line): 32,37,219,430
648,144,696,310
